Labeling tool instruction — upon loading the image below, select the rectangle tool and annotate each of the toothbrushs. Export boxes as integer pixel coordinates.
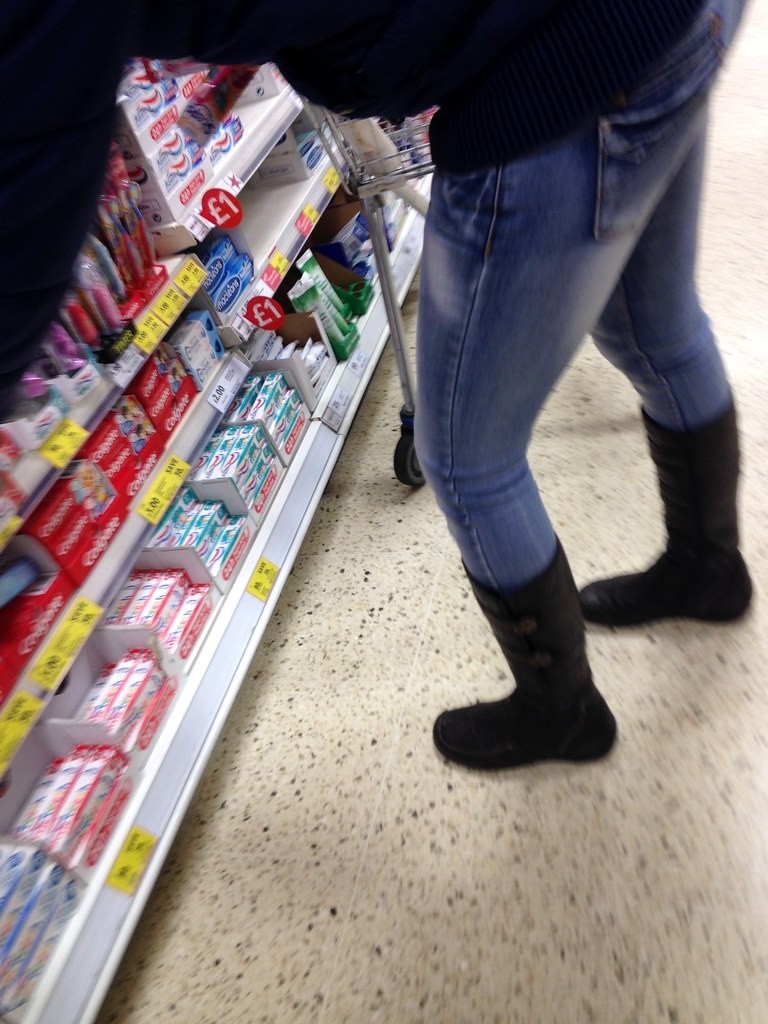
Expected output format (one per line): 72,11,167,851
18,145,156,417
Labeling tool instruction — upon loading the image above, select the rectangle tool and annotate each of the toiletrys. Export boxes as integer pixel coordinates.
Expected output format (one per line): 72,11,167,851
0,312,352,670
249,104,323,178
334,107,434,177
200,232,252,315
285,198,410,355
0,650,177,1021
111,57,285,226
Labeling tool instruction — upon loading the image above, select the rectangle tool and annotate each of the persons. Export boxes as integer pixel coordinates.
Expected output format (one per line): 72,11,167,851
1,0,758,771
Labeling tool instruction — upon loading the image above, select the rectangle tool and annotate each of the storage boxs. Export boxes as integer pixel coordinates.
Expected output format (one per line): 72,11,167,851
0,309,224,707
220,374,304,445
0,58,431,1024
188,423,276,504
74,649,178,750
146,485,247,572
99,567,218,659
116,58,404,314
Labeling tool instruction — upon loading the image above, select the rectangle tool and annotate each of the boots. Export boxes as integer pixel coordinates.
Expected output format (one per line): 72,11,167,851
431,535,617,772
578,400,753,626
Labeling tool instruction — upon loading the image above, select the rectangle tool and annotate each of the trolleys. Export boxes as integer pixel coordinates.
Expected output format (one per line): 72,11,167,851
302,93,438,487
